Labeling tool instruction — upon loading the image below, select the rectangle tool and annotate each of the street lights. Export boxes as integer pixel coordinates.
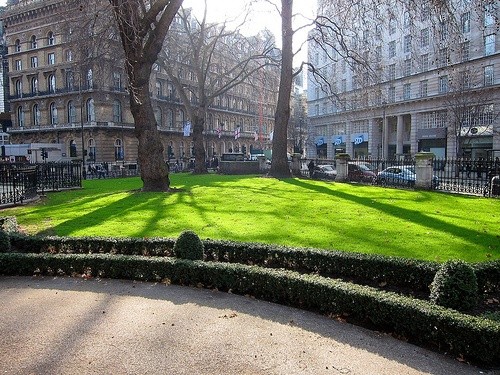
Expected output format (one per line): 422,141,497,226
382,100,387,170
183,86,207,158
65,69,86,177
298,115,304,167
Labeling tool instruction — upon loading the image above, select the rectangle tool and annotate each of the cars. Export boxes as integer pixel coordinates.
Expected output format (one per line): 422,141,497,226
313,165,336,180
348,161,381,184
377,165,440,188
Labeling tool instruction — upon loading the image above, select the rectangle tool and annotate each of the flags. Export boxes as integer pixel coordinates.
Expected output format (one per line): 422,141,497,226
270,132,273,142
250,125,261,142
234,126,241,140
215,126,222,138
183,120,190,136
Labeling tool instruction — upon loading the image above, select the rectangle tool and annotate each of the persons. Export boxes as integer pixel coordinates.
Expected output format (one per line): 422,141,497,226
308,159,316,180
88,161,110,181
263,156,272,178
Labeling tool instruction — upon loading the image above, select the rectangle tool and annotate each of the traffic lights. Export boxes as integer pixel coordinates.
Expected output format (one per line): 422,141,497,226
41,148,44,161
